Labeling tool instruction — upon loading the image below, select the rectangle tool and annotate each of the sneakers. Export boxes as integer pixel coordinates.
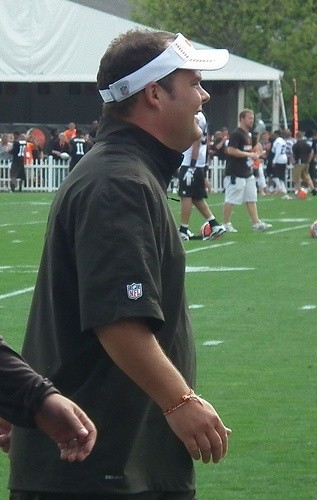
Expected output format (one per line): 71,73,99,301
178,230,192,242
203,224,226,240
221,222,238,233
252,220,272,232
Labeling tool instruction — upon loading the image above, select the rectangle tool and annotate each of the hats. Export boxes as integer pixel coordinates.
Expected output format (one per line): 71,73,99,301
99,32,229,103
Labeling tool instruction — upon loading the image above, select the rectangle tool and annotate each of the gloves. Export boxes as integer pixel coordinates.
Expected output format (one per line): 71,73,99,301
183,168,195,187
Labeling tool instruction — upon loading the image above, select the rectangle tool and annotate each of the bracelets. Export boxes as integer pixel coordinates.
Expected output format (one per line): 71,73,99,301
161,389,203,415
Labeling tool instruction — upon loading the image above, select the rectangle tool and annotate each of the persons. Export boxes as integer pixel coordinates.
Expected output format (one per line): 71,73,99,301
48,120,98,172
176,112,226,242
0,132,41,191
0,28,232,500
222,108,273,234
207,127,229,158
252,129,317,196
0,335,98,462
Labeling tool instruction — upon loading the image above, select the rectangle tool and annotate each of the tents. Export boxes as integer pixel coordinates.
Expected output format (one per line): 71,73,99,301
0,0,285,133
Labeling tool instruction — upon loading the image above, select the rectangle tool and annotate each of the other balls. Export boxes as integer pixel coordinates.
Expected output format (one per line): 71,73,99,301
311,222,317,238
294,188,307,199
201,223,221,240
